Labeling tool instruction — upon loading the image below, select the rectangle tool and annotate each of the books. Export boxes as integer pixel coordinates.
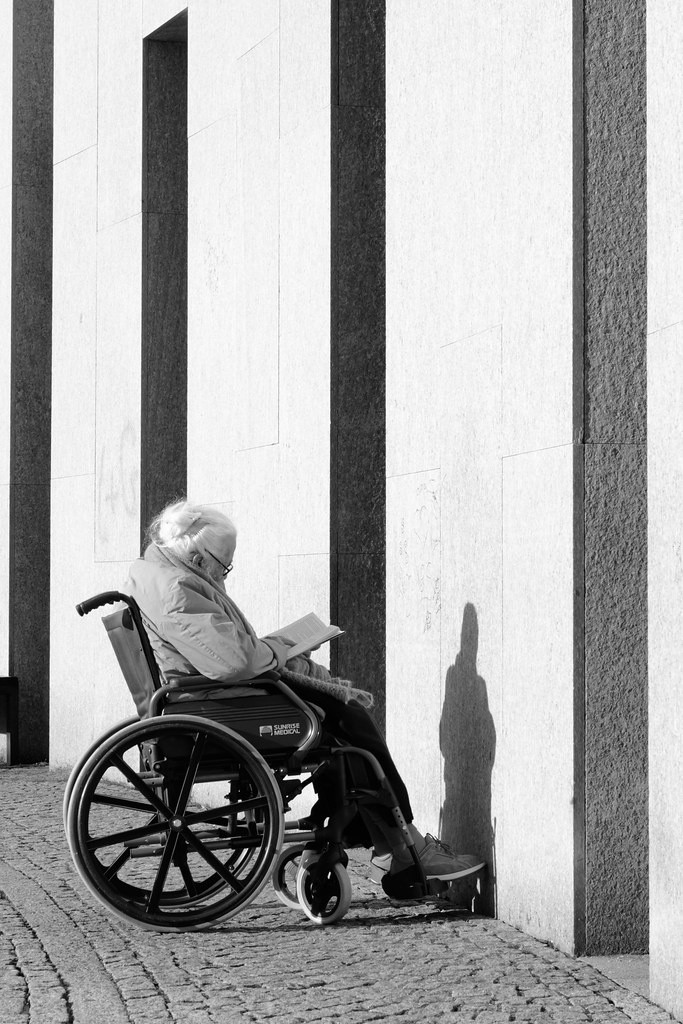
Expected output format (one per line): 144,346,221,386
260,611,347,661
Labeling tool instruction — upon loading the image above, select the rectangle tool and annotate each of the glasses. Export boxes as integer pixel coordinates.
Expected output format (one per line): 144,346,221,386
205,548,233,577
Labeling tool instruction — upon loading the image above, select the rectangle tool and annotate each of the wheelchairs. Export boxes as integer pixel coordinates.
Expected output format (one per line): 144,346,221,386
64,589,445,933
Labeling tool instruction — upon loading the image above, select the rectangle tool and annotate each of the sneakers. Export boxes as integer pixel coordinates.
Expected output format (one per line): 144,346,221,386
389,833,486,883
368,849,394,883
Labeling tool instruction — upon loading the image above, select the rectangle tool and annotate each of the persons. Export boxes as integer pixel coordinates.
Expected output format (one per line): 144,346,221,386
112,502,486,880
